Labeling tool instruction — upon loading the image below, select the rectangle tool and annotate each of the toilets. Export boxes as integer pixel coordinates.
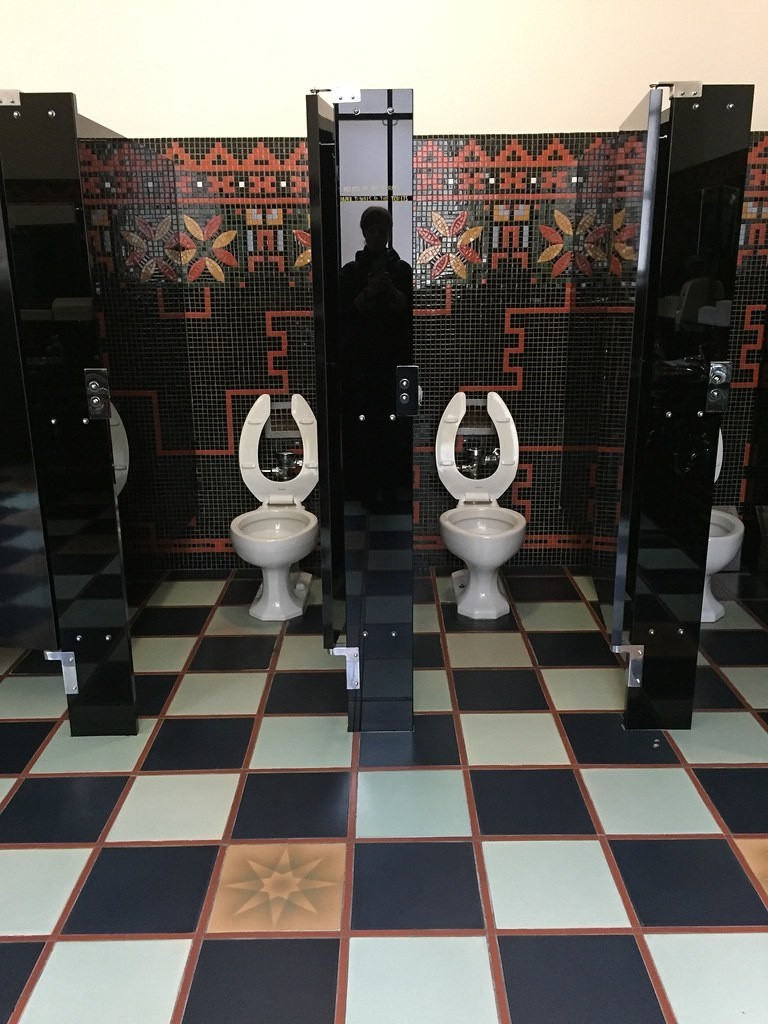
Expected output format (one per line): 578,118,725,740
436,392,526,618
700,429,745,622
230,393,318,621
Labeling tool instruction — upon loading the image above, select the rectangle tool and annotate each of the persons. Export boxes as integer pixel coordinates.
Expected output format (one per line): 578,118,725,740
336,206,413,508
